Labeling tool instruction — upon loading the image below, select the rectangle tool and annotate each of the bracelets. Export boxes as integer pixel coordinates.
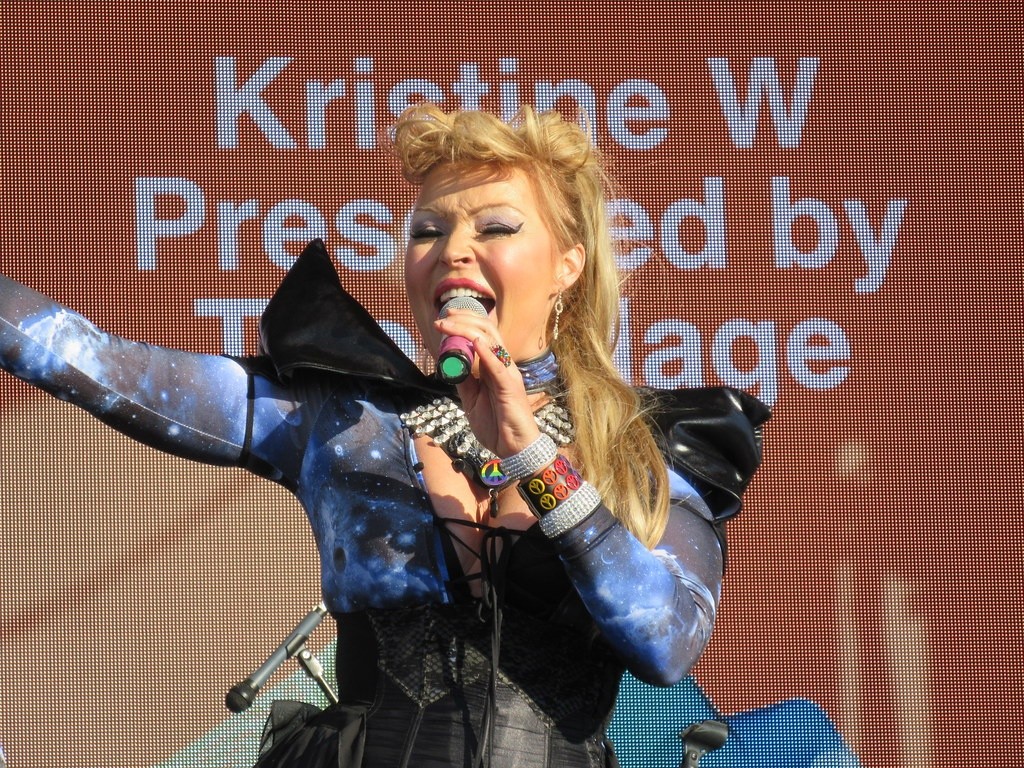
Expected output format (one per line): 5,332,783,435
491,430,602,540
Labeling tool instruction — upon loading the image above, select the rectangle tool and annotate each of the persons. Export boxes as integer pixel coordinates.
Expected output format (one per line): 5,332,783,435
2,92,775,765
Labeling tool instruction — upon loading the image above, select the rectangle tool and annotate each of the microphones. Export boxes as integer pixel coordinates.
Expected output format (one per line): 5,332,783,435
224,606,327,713
437,296,487,386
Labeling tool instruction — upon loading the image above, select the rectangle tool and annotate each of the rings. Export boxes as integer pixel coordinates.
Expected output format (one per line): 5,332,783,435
489,343,511,368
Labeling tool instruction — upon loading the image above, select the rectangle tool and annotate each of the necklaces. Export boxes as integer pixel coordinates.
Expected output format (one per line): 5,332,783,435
399,384,580,523
